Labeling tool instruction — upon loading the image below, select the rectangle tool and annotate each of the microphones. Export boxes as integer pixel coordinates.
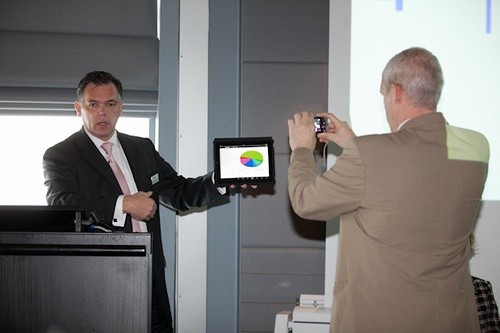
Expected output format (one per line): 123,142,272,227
108,160,110,164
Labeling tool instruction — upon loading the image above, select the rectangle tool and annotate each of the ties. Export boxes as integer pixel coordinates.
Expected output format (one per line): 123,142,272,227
100,142,140,232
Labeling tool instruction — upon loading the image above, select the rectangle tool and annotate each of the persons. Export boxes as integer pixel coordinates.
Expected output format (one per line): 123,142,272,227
469,235,500,333
42,72,256,333
287,47,490,333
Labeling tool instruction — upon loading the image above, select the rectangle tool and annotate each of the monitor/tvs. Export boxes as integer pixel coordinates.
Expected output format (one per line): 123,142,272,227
0,206,85,232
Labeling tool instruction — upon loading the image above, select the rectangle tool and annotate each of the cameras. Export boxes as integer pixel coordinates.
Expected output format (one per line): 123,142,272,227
314,116,326,135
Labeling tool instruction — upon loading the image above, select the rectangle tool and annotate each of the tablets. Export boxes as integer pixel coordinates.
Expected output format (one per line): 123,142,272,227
212,136,276,186
